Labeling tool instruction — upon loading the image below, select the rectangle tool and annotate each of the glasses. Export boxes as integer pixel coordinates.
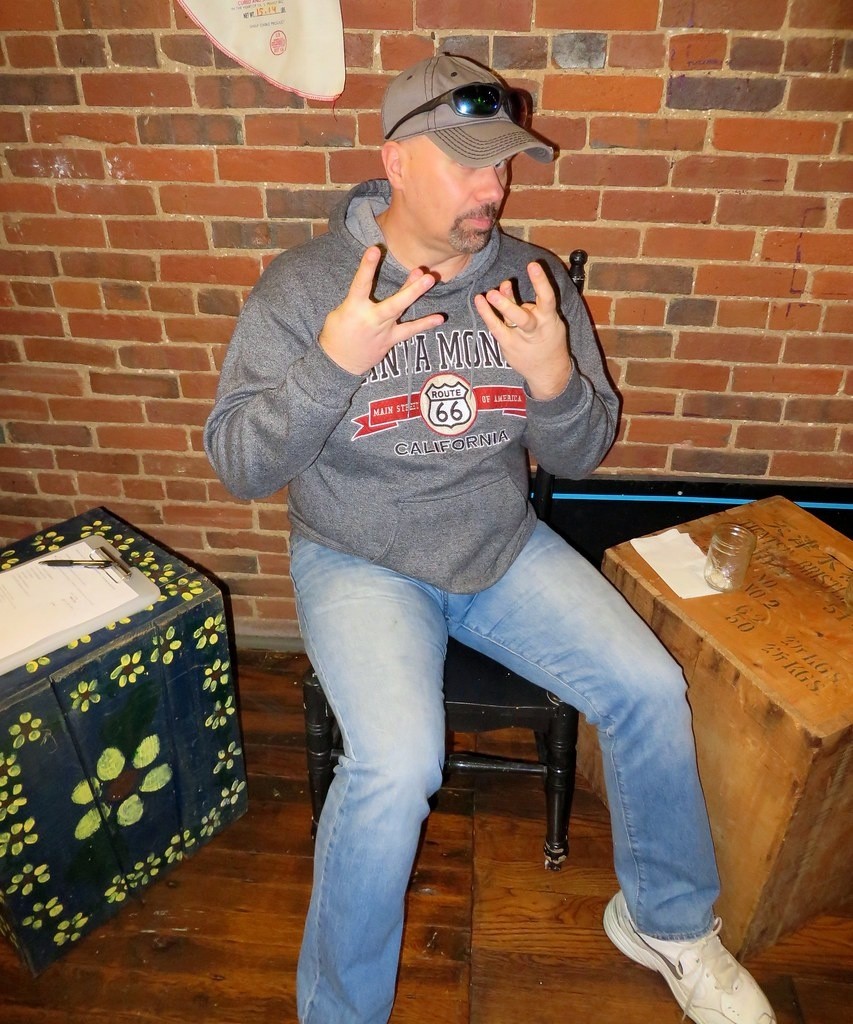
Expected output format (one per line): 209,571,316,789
385,83,528,139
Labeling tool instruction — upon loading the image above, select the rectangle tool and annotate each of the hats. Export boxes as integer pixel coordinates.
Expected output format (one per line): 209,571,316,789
382,52,555,168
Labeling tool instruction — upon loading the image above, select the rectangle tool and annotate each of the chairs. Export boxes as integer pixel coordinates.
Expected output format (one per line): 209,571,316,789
301,248,589,873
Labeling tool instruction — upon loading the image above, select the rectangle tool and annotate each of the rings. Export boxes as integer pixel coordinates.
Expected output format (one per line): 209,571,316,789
506,323,517,328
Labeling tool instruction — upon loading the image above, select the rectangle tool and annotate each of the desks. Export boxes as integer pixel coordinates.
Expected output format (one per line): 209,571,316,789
0,504,248,978
576,497,852,966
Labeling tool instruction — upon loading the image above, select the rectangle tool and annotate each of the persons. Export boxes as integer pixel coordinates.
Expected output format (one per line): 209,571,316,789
200,51,779,1024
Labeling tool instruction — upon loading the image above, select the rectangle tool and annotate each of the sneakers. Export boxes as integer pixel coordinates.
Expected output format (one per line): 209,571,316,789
602,887,777,1024
